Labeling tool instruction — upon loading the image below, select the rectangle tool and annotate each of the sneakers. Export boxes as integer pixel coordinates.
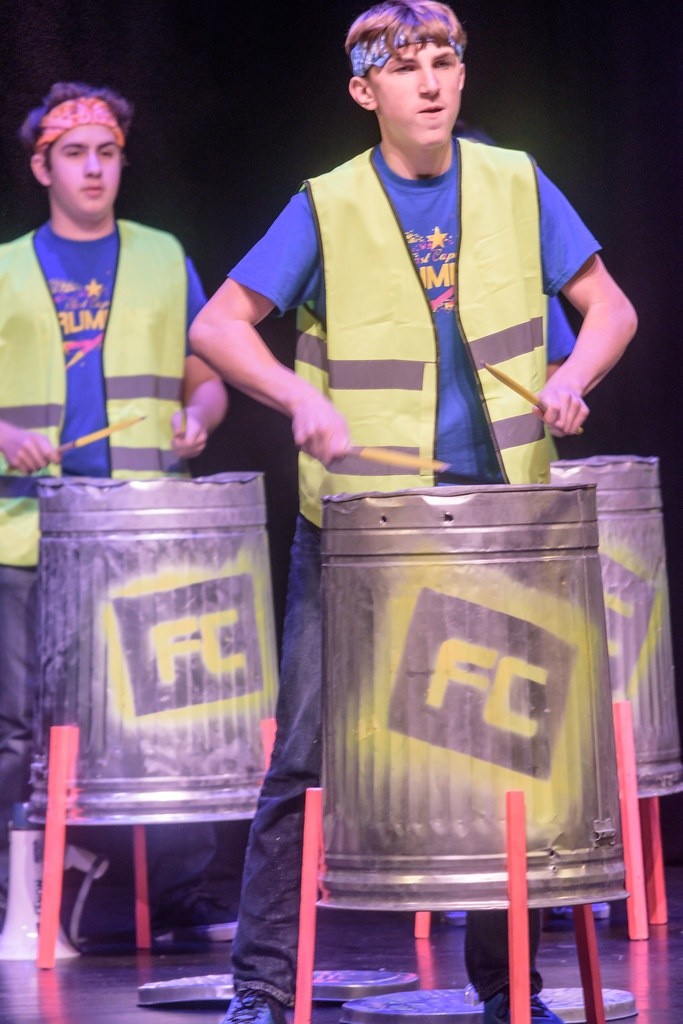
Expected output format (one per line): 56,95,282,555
222,990,284,1024
483,992,562,1024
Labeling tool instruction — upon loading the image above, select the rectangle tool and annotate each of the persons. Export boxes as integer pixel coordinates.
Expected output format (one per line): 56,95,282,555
0,80,241,950
189,0,637,1024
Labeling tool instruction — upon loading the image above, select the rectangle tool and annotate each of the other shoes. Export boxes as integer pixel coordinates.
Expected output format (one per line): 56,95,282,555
175,904,239,942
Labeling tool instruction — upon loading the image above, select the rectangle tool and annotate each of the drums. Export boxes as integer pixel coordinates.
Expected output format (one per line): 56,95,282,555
547,451,683,802
22,469,286,832
309,478,635,916
